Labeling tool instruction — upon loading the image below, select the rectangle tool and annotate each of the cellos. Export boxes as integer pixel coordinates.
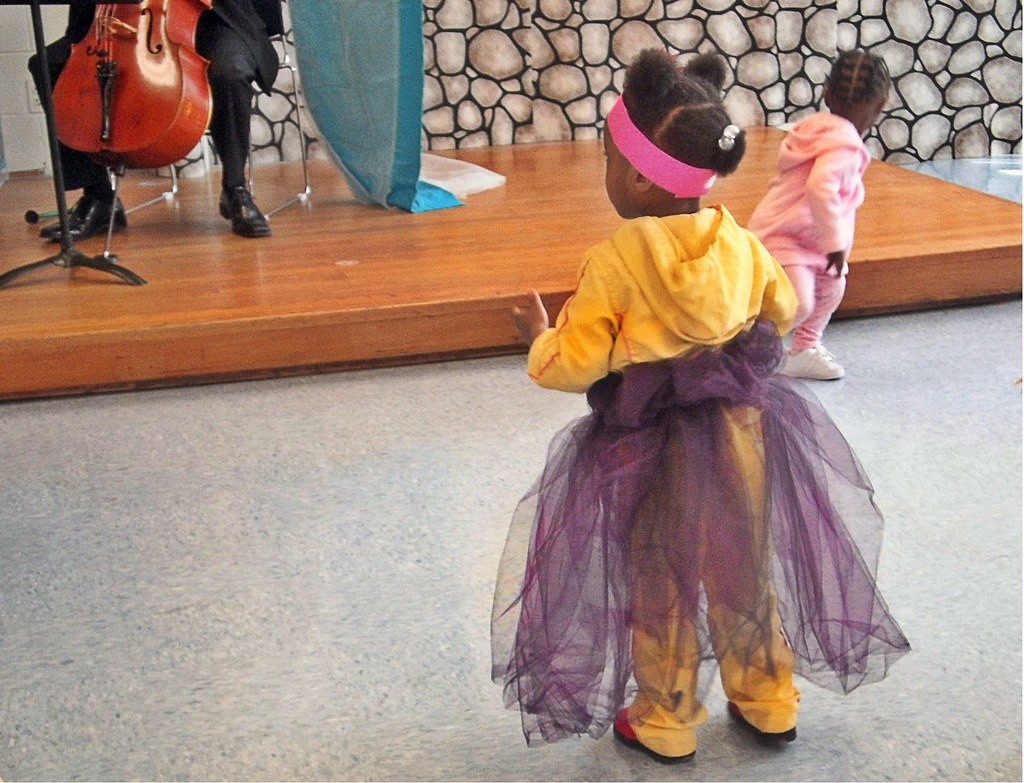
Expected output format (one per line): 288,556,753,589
52,0,214,259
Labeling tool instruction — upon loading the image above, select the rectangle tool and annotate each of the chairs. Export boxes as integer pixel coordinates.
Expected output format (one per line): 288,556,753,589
109,0,309,218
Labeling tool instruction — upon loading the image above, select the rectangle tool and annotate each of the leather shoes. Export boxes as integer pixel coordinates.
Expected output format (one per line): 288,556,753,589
218,184,272,236
39,191,128,241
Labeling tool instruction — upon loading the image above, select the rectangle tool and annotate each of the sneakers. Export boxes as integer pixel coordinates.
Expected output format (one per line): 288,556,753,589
777,344,844,380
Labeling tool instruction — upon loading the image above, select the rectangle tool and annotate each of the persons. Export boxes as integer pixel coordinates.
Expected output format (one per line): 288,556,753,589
28,1,284,241
491,47,911,761
747,50,890,379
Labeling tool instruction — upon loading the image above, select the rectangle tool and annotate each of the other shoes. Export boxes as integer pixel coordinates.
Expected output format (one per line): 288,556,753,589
728,699,797,745
613,707,696,764
